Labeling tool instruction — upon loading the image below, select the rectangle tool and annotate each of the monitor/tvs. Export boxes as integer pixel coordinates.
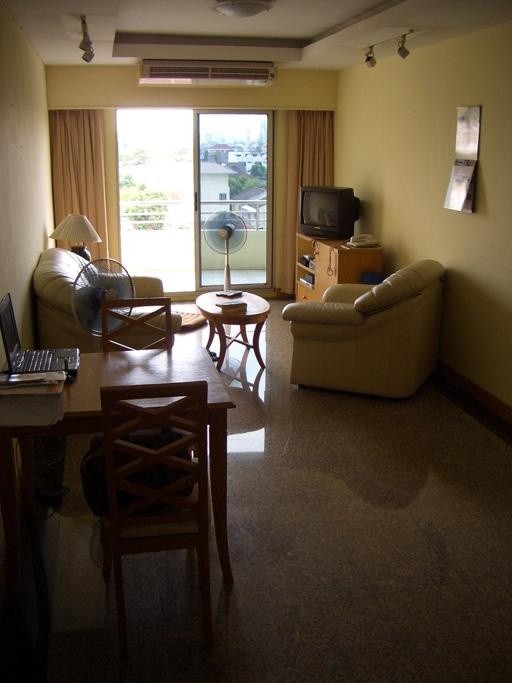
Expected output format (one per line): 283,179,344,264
300,185,360,239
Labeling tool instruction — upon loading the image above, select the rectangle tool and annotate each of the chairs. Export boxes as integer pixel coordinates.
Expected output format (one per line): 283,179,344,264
98,381,213,667
32,247,183,350
281,260,445,397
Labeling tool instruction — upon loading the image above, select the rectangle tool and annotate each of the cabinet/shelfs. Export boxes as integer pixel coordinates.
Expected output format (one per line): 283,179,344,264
294,233,382,304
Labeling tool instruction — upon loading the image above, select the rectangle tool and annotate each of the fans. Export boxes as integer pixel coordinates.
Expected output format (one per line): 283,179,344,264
203,212,248,297
71,258,135,338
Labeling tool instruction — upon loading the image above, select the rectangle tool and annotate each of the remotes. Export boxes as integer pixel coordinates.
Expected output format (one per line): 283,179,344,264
8,370,67,384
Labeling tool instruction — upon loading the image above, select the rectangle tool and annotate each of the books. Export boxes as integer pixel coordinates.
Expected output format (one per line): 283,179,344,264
216,301,247,314
0,370,68,427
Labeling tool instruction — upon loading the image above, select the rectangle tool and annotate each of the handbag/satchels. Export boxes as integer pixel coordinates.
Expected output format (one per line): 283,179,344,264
79,431,194,518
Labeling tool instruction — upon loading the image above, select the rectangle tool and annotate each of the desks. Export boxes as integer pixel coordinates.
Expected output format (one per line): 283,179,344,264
0,346,236,610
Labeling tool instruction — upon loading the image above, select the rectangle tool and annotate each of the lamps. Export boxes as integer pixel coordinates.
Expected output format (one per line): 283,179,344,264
79,19,95,62
49,213,103,260
364,29,416,68
209,0,273,20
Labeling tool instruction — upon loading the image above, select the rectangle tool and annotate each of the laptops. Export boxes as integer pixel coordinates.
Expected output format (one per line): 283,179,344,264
0,292,80,378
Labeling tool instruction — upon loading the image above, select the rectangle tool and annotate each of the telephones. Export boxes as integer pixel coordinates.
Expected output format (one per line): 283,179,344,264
347,234,379,248
